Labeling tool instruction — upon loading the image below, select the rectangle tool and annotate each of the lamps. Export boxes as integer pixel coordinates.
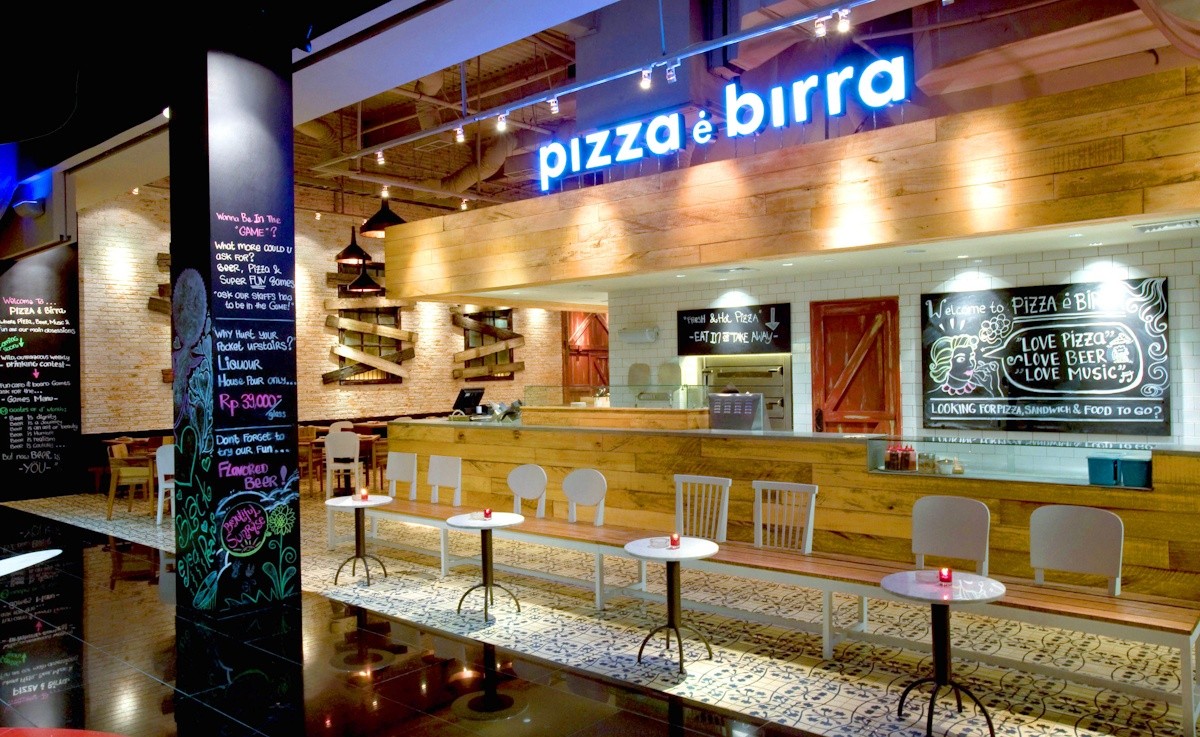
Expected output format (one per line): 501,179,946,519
639,56,681,89
315,185,407,292
814,5,851,37
546,93,560,115
497,109,511,131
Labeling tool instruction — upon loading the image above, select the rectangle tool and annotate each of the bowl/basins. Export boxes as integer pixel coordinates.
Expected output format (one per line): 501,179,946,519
570,402,586,408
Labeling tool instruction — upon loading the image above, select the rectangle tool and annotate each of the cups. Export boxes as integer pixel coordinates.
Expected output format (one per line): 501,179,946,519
670,534,681,550
361,489,368,501
938,568,953,586
476,406,482,414
483,507,492,521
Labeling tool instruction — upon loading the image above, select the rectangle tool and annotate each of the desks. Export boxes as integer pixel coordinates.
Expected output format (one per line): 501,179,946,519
624,537,719,672
880,570,1006,737
324,494,392,586
103,437,151,497
446,512,525,622
310,435,381,494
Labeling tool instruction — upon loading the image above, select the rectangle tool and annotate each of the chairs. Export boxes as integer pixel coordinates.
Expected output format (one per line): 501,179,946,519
107,424,389,524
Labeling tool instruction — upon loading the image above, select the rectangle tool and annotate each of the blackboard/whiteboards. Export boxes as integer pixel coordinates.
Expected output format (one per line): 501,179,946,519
677,302,792,355
920,276,1172,437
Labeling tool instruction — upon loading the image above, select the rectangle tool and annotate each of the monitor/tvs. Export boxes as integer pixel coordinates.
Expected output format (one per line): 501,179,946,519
452,386,485,415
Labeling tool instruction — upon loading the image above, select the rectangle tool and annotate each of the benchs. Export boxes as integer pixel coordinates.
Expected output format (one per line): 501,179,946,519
348,450,1200,737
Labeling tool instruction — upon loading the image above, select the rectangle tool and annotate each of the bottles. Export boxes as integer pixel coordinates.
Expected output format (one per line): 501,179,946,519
884,443,916,471
